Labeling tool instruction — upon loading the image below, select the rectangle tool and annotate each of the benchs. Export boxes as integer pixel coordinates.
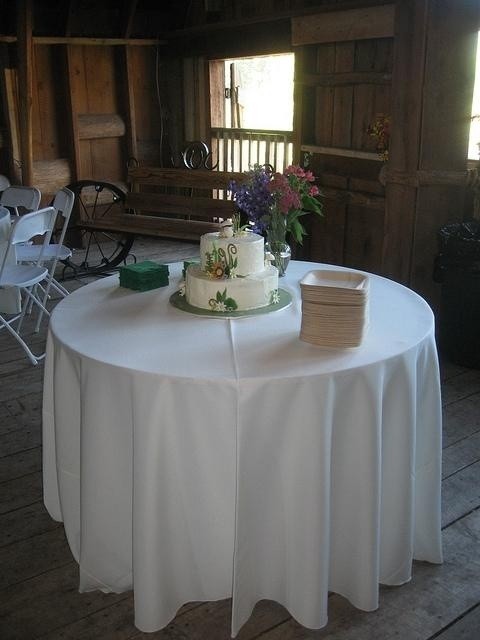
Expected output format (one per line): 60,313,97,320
49,141,253,282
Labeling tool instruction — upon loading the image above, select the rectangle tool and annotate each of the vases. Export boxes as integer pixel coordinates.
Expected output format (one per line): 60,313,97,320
262,230,292,278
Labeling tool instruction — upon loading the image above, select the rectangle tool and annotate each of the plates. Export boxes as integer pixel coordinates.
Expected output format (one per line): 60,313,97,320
297,270,370,349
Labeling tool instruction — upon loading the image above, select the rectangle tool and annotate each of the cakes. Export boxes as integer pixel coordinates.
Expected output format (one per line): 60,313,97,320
185,219,279,312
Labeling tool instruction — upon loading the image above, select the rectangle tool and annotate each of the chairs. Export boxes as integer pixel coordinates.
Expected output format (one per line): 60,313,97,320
0,176,79,367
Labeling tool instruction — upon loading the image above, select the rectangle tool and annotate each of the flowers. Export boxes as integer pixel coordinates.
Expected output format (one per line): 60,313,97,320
226,154,328,272
